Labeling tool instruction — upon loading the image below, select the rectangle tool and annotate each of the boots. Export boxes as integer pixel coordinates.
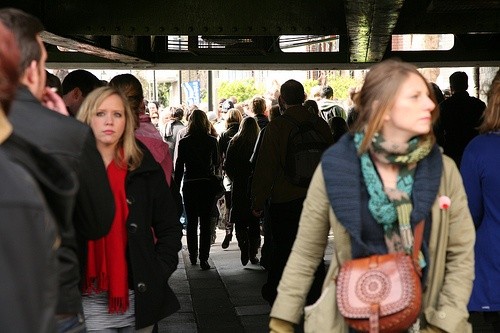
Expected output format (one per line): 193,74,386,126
222,209,233,248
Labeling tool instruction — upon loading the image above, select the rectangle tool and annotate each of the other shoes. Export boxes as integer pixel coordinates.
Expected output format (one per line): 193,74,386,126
199,261,210,268
190,256,196,264
250,256,259,264
241,250,250,267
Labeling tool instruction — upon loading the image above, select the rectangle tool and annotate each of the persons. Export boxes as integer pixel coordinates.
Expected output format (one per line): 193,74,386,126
0,8,500,333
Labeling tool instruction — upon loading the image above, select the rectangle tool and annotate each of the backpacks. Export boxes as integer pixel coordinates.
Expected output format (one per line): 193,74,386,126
281,113,330,186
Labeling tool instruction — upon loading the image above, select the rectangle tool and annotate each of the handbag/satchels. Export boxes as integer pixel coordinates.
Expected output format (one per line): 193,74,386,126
336,248,421,333
211,176,226,198
